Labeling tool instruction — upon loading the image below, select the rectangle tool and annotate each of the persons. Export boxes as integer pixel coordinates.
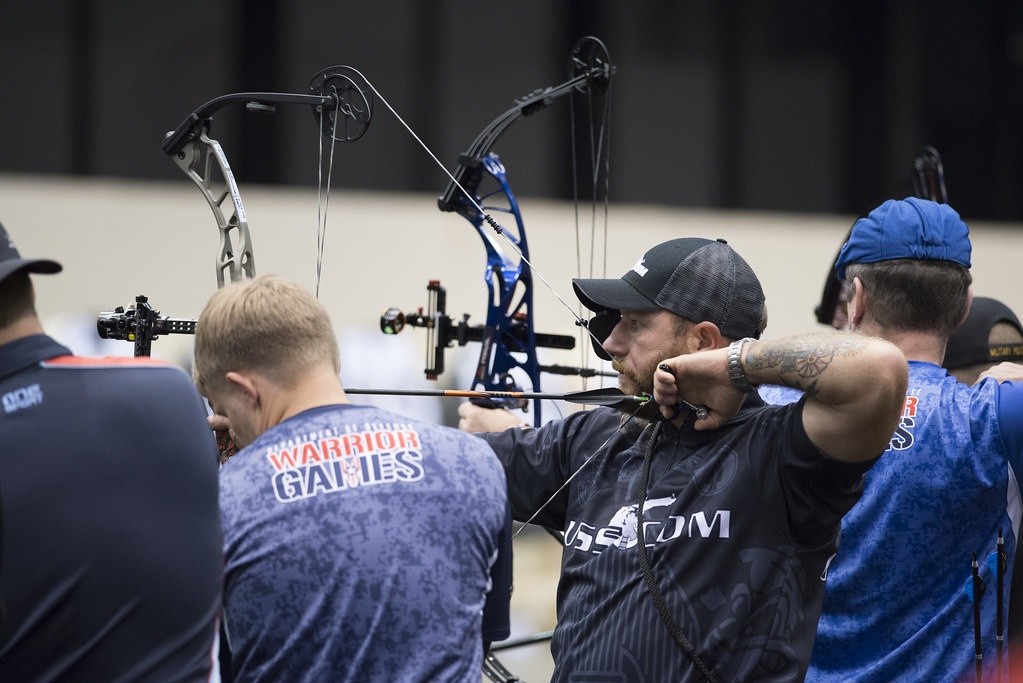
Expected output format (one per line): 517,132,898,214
207,238,909,683
459,197,1023,683
941,297,1023,683
0,224,224,683
192,274,507,683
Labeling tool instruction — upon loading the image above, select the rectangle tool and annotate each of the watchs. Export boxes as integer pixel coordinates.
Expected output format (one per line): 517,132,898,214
727,337,762,393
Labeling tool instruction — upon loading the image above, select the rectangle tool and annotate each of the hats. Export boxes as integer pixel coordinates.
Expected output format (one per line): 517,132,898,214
0,221,62,287
571,236,764,341
835,194,973,280
943,298,1022,372
